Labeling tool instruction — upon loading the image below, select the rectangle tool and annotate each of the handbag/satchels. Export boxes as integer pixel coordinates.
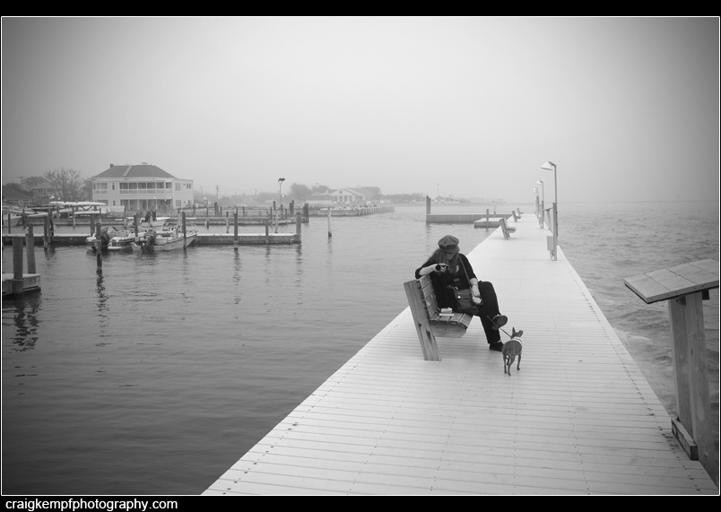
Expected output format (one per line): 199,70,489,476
450,288,480,315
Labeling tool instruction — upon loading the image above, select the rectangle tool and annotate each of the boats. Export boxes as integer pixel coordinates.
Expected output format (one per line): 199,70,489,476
85,228,199,254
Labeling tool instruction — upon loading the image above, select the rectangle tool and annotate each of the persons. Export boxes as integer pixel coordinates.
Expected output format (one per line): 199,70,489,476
415,234,508,352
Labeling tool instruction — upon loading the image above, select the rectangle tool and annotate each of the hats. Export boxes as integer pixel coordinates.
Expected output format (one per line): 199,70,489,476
437,235,460,254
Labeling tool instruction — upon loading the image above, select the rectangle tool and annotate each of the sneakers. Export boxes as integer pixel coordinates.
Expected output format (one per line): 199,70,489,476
490,341,504,352
490,314,507,330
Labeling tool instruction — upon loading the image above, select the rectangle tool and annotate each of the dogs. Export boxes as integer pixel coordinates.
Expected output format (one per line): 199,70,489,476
502,326,523,376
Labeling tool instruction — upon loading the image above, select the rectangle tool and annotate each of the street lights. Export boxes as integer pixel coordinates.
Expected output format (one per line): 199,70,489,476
533,161,556,261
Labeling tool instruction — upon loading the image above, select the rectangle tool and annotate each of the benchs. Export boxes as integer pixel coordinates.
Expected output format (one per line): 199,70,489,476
404,275,472,361
500,218,516,240
